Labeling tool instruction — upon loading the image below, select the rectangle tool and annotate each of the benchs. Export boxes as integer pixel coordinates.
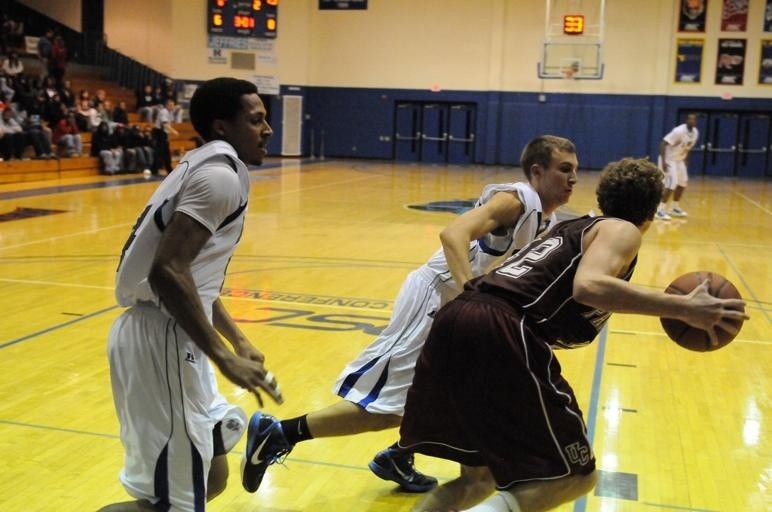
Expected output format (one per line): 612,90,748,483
1,41,205,184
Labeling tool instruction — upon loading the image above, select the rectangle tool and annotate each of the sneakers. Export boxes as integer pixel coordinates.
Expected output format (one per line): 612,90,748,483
368,440,438,492
668,205,687,217
655,208,672,220
237,409,295,494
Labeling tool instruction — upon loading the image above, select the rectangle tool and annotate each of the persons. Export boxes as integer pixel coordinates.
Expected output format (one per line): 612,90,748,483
99,76,286,512
0,20,184,175
398,156,750,512
658,114,700,220
239,134,579,494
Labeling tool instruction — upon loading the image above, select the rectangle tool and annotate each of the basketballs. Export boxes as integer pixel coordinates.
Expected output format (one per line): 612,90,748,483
660,272,744,352
